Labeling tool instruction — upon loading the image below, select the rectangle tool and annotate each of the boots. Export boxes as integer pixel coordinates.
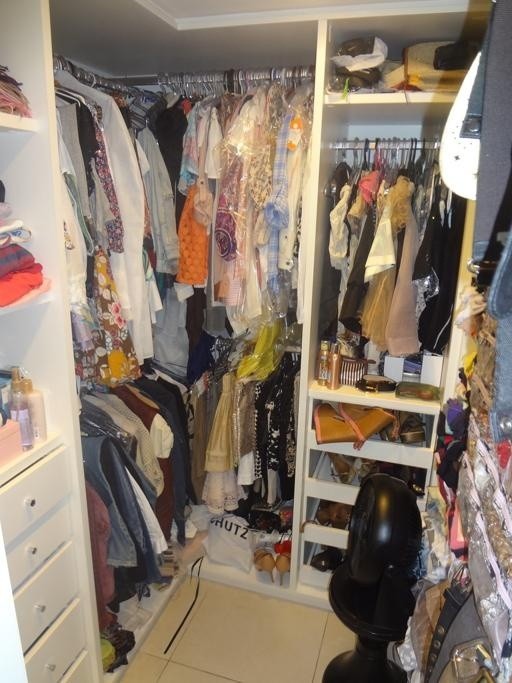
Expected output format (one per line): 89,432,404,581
314,403,426,444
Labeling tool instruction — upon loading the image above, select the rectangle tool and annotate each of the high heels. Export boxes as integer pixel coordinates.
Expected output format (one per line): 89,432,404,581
254,548,275,581
275,554,290,585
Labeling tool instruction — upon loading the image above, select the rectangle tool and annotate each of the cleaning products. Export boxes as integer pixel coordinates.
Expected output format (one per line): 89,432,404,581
9,366,46,451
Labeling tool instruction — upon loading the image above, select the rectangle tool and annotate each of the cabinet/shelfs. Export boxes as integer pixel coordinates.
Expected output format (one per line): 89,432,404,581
0,0,512,683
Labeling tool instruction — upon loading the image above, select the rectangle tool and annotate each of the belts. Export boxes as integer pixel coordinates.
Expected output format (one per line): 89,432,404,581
422,565,496,683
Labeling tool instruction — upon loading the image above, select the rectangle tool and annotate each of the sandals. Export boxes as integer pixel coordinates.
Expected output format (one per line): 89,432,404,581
325,452,425,497
310,502,352,572
